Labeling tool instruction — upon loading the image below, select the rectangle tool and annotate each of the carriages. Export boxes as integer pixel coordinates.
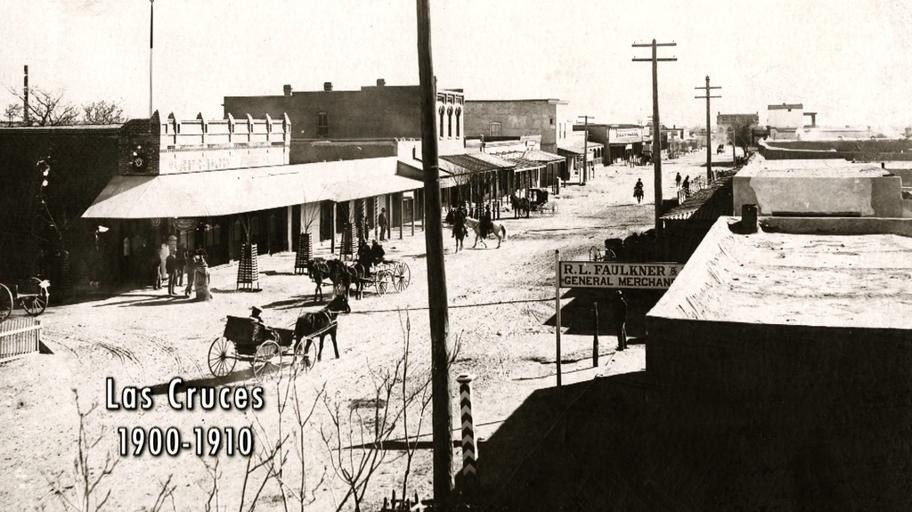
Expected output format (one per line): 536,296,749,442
207,292,353,381
510,188,558,218
307,255,412,297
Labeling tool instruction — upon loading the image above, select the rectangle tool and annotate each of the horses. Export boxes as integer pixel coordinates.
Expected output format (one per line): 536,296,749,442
509,192,529,219
294,293,351,367
445,208,505,250
304,257,353,304
453,209,466,254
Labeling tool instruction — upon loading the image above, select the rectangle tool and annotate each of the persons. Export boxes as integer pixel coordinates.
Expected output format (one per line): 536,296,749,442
369,240,385,259
150,245,193,297
480,204,491,240
682,176,689,194
451,204,469,238
632,178,644,199
357,240,372,276
364,216,370,240
378,206,388,241
249,305,263,323
675,172,682,187
